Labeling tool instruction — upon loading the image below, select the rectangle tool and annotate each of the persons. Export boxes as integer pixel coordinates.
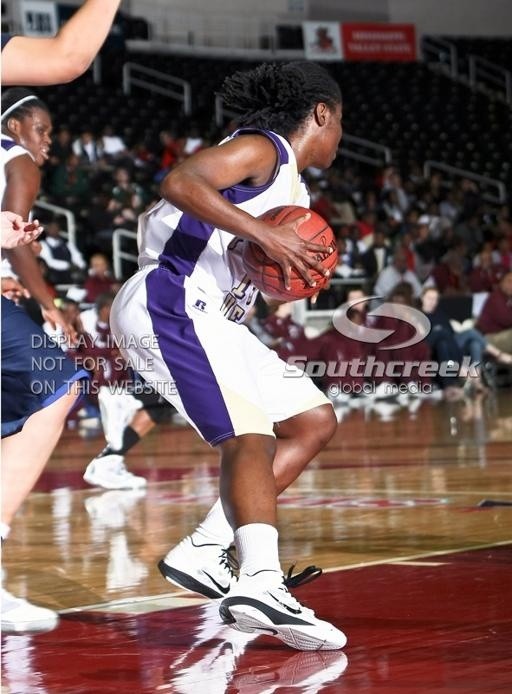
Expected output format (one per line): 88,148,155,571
108,59,349,654
84,383,178,491
1,1,123,89
1,125,512,436
1,87,94,635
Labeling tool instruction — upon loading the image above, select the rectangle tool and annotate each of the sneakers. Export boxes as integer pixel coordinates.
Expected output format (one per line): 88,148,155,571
158,535,240,606
219,566,347,651
98,386,142,452
83,454,147,489
327,382,444,405
2,589,58,636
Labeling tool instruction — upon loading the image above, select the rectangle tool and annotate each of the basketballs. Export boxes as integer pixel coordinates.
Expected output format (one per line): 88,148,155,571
241,205,343,300
